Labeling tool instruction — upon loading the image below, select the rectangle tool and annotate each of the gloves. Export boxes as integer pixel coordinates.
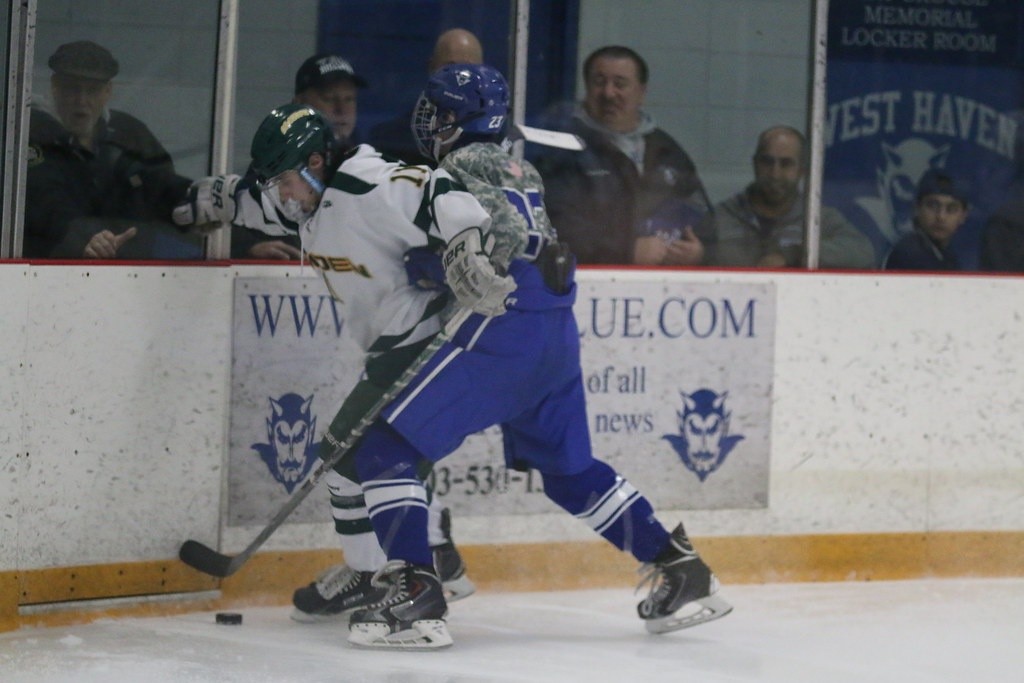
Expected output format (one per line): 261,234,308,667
439,227,517,317
171,173,240,234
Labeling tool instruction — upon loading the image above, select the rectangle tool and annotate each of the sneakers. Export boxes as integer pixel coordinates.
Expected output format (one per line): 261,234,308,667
348,559,453,649
637,523,732,637
427,508,476,603
292,561,392,623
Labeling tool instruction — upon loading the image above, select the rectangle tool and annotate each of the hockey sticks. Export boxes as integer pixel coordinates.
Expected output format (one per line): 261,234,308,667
177,262,507,579
500,122,588,155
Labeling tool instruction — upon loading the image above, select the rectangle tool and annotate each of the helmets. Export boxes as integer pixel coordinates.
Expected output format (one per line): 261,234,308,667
250,103,335,189
410,64,511,161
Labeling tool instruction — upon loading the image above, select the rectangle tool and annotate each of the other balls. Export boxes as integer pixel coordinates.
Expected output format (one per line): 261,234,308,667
215,611,243,625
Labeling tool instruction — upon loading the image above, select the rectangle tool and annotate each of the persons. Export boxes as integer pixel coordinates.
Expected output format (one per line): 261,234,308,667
369,28,483,171
347,63,721,624
230,54,371,260
22,40,193,260
172,104,467,616
702,125,875,269
534,45,717,266
885,168,977,270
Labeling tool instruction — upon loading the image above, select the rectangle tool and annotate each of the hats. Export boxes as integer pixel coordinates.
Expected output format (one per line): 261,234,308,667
294,54,367,93
49,39,120,81
916,168,968,204
